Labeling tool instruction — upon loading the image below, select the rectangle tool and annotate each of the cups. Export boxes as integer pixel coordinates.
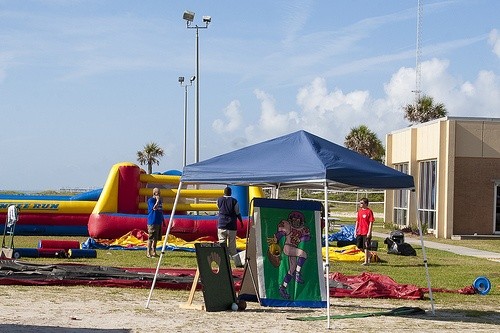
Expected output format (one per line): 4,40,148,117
232,301,247,311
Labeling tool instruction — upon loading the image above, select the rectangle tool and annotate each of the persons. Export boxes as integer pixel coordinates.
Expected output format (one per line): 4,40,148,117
215,188,244,256
146,187,166,259
354,197,377,266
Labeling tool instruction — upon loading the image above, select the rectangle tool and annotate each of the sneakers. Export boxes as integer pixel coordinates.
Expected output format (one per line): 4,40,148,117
367,254,373,263
146,255,151,258
152,253,159,257
361,262,369,265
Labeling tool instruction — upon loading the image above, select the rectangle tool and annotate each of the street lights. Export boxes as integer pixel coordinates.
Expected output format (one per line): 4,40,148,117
178,76,195,169
183,10,211,216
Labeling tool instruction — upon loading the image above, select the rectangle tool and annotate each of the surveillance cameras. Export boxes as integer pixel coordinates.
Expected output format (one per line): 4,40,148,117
190,76,195,81
202,16,212,22
